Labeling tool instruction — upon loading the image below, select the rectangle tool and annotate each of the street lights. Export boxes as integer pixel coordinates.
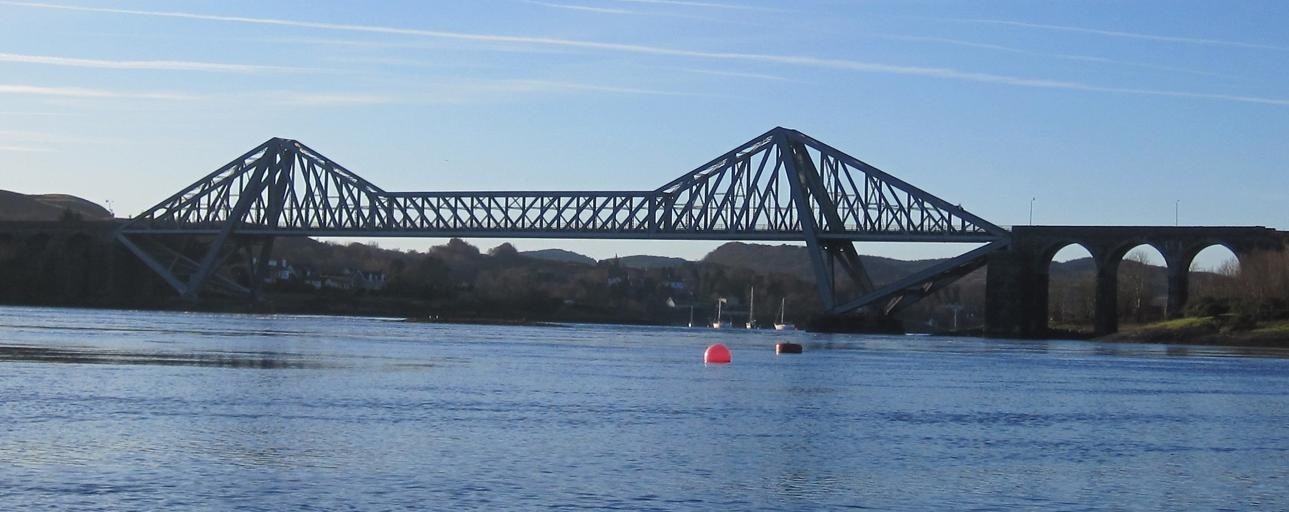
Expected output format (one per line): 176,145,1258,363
1029,197,1035,226
1175,199,1180,226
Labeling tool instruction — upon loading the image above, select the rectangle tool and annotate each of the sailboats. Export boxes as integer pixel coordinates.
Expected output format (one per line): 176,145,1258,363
686,283,796,331
